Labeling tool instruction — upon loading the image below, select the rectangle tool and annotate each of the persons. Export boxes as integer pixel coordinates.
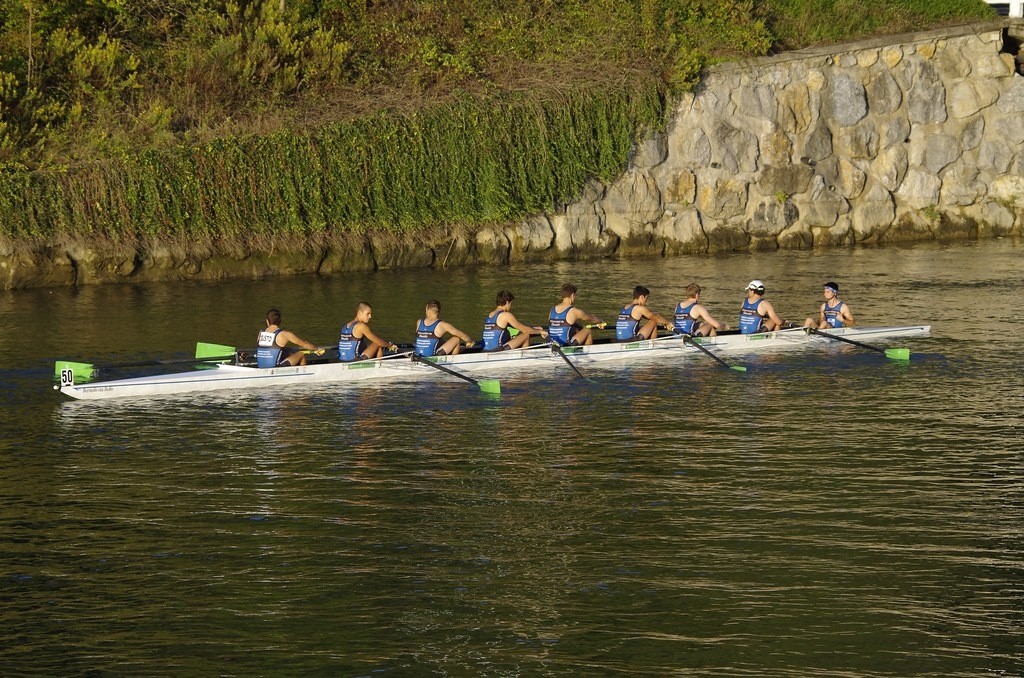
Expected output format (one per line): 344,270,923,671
255,308,320,368
615,285,672,343
338,302,398,361
738,280,786,334
415,299,473,356
674,282,729,336
548,283,605,347
482,289,548,353
804,282,856,329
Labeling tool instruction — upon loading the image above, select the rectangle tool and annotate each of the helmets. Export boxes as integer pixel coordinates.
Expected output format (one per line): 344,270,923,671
745,279,765,291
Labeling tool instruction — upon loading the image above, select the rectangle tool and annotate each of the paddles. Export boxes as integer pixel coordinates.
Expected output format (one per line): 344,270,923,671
194,341,326,370
594,325,668,330
552,341,599,385
666,323,748,372
293,344,399,354
505,326,550,338
411,354,501,395
805,327,911,361
389,343,469,348
762,313,801,329
537,323,608,331
54,352,245,385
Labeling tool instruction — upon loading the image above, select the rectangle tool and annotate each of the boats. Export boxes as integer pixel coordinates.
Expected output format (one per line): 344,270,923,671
55,325,935,405
